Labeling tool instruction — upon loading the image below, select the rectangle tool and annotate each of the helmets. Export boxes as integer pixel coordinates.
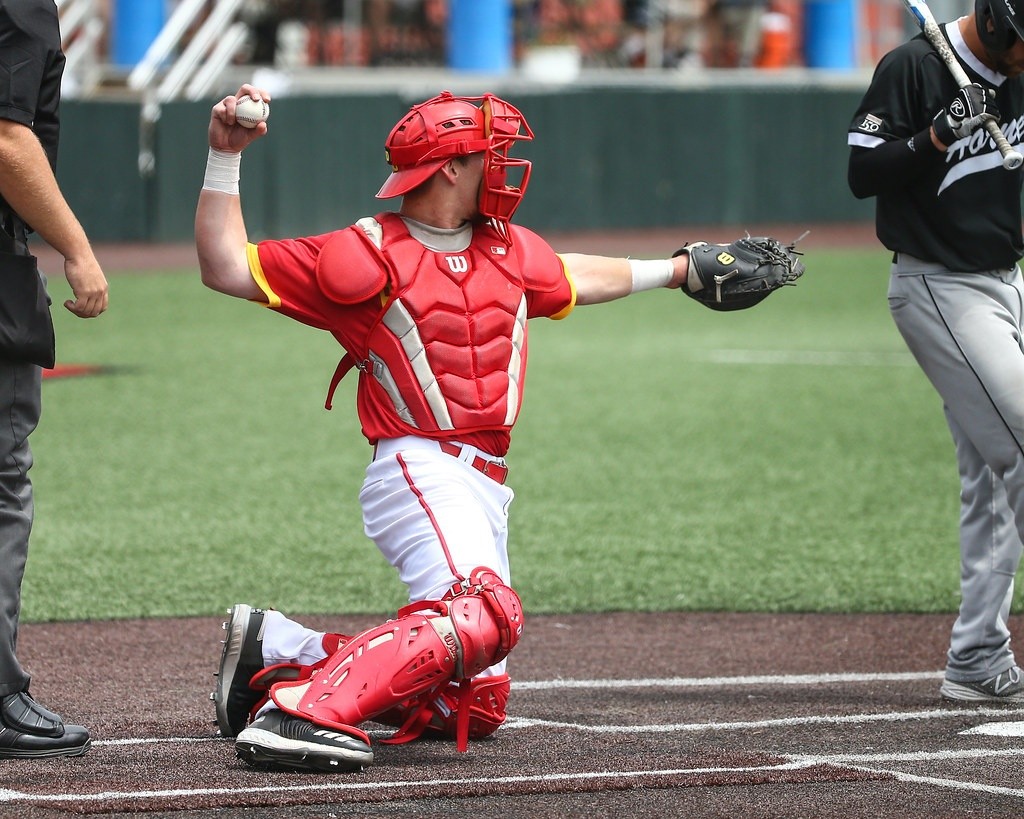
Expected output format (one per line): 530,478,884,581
975,0,1024,52
375,91,489,201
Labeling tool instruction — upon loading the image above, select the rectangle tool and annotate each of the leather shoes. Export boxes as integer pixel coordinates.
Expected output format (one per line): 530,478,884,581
0,692,92,758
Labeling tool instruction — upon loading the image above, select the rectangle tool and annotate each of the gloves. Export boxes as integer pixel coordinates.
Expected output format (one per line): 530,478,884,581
932,83,1001,146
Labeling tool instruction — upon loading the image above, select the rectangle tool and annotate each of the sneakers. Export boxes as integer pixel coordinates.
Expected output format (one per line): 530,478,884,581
940,666,1024,702
210,603,267,738
235,708,374,775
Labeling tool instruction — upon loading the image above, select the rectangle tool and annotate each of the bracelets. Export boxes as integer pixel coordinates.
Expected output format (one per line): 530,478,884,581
628,259,674,293
202,146,240,195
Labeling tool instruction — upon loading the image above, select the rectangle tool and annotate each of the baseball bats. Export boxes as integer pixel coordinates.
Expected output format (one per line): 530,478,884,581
901,0,1023,171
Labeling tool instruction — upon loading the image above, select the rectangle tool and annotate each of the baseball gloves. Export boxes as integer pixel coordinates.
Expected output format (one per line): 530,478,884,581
671,229,807,312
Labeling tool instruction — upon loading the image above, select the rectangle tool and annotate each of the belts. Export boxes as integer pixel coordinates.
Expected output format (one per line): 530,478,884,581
439,440,508,484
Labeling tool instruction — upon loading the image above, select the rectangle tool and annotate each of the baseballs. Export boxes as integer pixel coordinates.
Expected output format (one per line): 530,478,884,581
234,94,271,129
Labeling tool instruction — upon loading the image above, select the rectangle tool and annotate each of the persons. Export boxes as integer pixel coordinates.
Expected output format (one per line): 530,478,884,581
0,0,109,760
847,0,1024,703
194,84,806,773
